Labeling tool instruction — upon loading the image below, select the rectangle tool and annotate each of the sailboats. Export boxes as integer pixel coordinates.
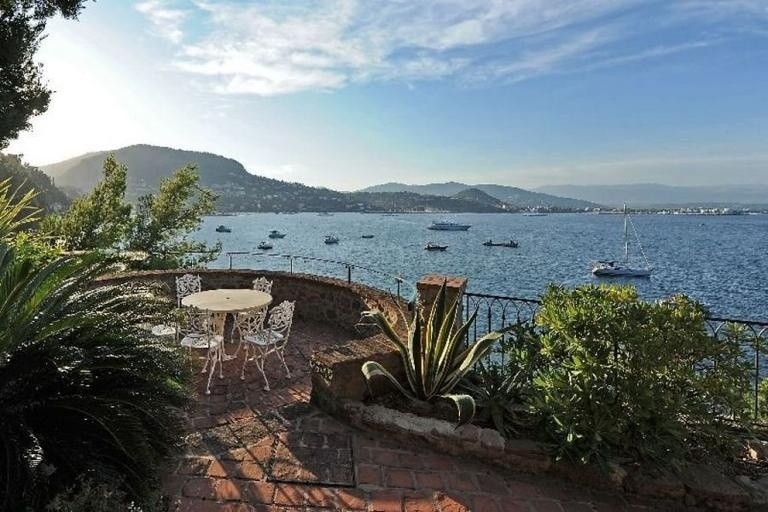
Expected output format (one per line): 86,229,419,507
593,206,654,277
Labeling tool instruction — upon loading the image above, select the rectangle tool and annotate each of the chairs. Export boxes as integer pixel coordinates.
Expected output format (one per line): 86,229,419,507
150,272,296,396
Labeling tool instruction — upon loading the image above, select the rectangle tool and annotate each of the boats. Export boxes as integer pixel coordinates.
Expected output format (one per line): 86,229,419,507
424,243,448,251
268,230,287,239
323,232,374,243
427,220,472,230
215,226,232,233
484,239,518,248
257,241,271,249
